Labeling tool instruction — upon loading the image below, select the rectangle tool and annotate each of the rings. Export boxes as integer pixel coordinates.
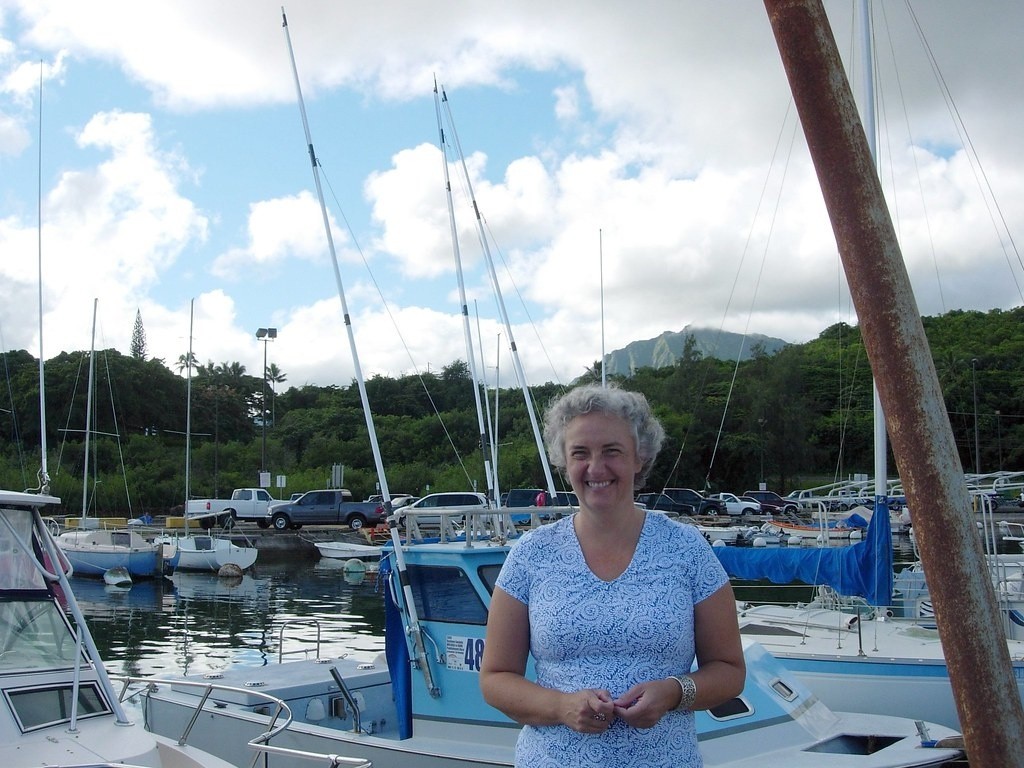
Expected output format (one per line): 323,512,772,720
596,713,606,720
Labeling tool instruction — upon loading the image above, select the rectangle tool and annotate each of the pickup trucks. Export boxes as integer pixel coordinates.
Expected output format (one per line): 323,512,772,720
266,489,386,531
184,488,274,530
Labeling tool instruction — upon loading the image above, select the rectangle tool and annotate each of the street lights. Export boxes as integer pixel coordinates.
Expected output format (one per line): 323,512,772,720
255,328,278,472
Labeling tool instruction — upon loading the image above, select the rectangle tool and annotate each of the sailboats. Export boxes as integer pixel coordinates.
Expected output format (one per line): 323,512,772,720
126,0,1024,766
50,298,180,585
150,299,259,573
1,59,378,768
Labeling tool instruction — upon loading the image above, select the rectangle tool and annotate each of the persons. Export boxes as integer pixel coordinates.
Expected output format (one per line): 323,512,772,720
479,385,745,768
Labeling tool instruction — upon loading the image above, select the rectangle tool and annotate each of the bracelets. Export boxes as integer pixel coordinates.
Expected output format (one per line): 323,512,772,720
665,673,697,713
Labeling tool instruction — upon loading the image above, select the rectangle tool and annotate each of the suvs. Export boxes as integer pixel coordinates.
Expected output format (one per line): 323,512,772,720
393,492,493,529
501,488,804,525
